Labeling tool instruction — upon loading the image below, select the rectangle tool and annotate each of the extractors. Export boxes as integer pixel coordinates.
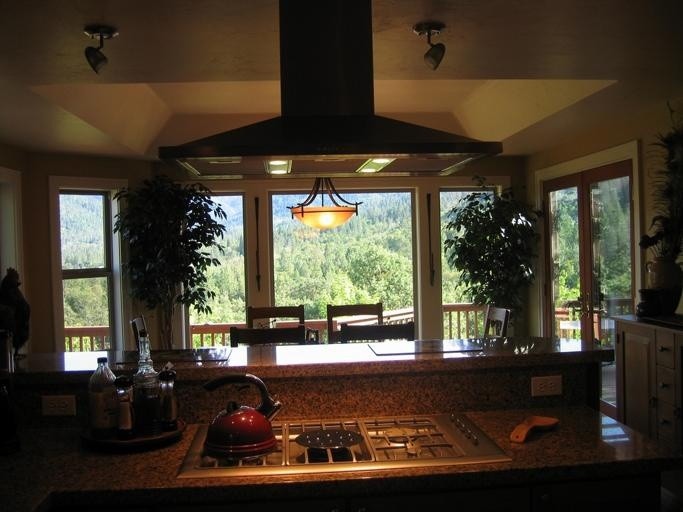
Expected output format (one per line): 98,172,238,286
158,1,504,181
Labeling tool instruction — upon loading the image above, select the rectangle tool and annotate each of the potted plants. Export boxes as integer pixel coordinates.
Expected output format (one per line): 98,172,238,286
637,92,682,312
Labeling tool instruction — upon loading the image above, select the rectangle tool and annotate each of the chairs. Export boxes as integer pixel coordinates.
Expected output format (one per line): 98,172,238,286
482,303,511,338
128,313,148,350
228,302,416,347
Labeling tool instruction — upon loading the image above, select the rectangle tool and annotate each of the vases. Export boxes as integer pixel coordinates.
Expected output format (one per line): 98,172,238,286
635,287,659,318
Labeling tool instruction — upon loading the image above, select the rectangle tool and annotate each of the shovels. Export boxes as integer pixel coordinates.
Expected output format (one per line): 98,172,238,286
510,415,560,443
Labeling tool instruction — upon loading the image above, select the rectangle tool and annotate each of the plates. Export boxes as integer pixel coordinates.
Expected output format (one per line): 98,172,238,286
78,417,188,450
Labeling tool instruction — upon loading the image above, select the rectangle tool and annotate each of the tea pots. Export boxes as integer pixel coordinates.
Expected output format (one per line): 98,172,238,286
203,372,283,458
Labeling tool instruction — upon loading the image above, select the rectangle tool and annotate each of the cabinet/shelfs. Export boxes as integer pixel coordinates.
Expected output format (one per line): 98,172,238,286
608,312,682,452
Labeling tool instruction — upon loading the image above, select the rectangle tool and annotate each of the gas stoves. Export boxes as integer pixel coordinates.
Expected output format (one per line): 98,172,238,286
175,412,513,480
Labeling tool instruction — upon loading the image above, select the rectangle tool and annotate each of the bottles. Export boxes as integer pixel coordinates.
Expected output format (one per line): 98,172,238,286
87,358,120,441
157,368,178,433
132,329,159,437
113,377,137,441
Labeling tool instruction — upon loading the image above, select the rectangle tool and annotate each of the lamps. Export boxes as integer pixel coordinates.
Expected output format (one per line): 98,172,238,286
79,23,120,75
413,20,448,73
284,176,364,233
